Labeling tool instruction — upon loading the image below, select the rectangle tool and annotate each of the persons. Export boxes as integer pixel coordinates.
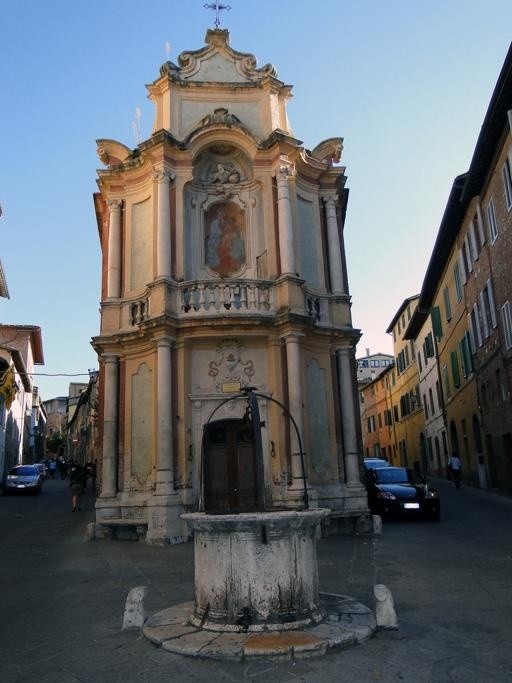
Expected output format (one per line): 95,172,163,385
448,452,461,487
206,206,244,275
47,455,96,511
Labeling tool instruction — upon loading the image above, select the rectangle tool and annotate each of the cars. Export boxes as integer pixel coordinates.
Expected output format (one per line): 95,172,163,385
33,454,66,480
368,465,442,523
364,457,393,470
4,463,43,496
